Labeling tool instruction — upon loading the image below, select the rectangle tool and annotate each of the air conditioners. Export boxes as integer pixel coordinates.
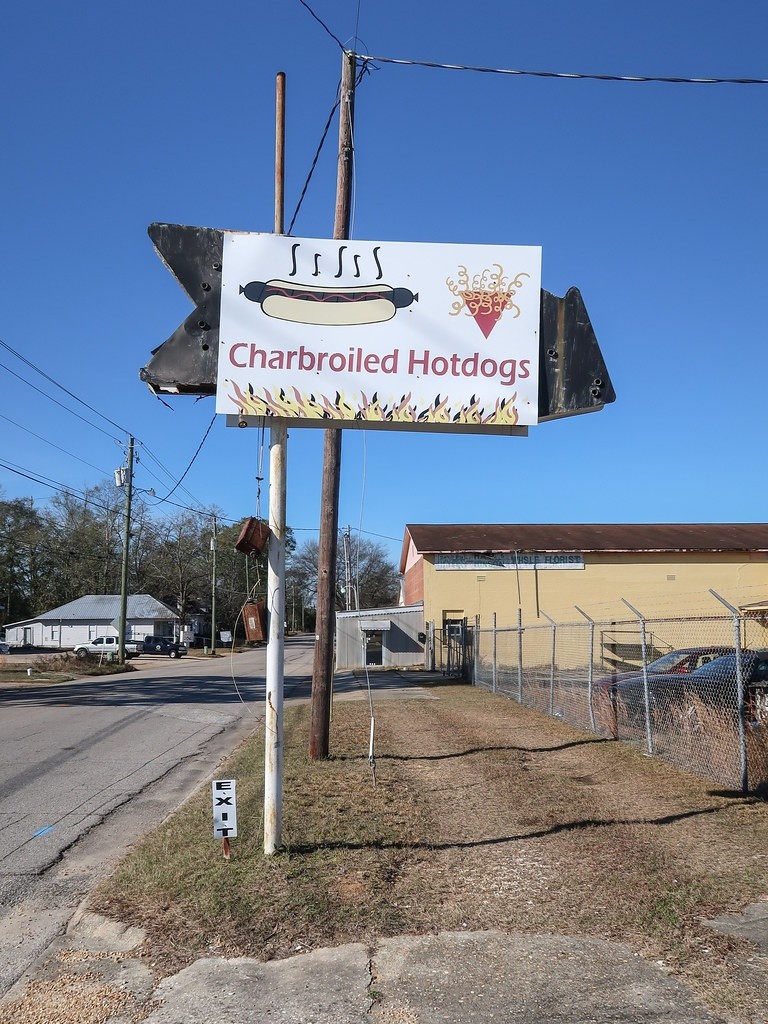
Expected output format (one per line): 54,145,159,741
446,624,462,637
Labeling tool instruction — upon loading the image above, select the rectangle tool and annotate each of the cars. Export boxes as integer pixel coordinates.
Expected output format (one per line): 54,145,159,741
592,646,759,706
609,652,768,730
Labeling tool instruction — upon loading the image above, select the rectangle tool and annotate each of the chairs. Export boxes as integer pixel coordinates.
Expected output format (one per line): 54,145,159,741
702,656,711,667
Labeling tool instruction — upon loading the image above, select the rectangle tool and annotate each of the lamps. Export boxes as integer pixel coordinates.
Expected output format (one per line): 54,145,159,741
238,414,247,428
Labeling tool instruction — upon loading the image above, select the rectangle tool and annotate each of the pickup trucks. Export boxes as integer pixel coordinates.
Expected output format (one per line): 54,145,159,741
125,636,187,658
73,636,144,659
